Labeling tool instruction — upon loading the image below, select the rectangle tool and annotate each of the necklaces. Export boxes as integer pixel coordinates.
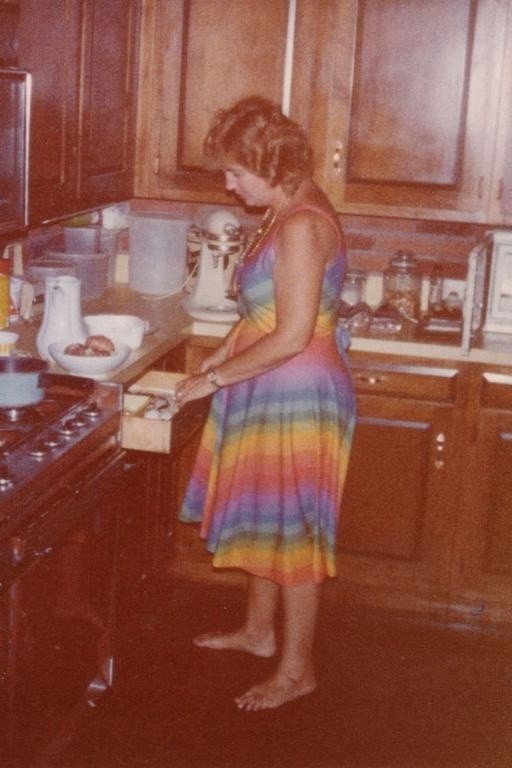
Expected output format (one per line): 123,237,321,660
238,195,287,264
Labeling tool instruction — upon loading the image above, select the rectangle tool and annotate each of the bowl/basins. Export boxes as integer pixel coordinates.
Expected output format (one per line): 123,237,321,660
81,313,149,350
48,339,131,383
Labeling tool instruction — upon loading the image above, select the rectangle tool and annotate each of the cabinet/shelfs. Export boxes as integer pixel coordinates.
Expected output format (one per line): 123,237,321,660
1,1,138,244
0,343,188,767
176,337,511,629
137,1,511,213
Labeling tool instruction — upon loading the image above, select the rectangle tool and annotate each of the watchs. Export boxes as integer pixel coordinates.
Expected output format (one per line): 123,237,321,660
207,368,222,389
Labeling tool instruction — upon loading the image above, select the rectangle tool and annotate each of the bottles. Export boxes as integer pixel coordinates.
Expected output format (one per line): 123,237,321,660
342,270,367,307
382,250,423,326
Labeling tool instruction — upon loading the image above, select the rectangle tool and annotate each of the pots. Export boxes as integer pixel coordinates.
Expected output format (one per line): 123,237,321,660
0,355,95,409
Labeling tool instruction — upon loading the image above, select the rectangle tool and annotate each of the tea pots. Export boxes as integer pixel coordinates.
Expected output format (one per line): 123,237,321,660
35,274,89,364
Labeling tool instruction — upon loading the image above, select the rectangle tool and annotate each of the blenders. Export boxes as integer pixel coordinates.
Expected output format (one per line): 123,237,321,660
180,204,246,323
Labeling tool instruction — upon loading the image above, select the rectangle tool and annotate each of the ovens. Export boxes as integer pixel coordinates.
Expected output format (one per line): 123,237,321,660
0,443,125,768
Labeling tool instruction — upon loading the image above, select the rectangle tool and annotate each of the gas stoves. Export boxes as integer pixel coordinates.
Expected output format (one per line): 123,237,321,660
0,382,122,502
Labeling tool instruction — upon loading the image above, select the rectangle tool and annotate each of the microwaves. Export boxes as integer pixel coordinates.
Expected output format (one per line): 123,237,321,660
460,229,511,357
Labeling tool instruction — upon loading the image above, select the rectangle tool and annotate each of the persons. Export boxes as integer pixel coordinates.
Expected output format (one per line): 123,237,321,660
176,95,359,712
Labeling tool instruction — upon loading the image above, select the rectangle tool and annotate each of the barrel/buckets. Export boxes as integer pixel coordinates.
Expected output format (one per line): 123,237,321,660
126,209,191,297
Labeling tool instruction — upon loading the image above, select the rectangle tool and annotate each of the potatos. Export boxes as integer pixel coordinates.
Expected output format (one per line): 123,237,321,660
86,335,114,353
